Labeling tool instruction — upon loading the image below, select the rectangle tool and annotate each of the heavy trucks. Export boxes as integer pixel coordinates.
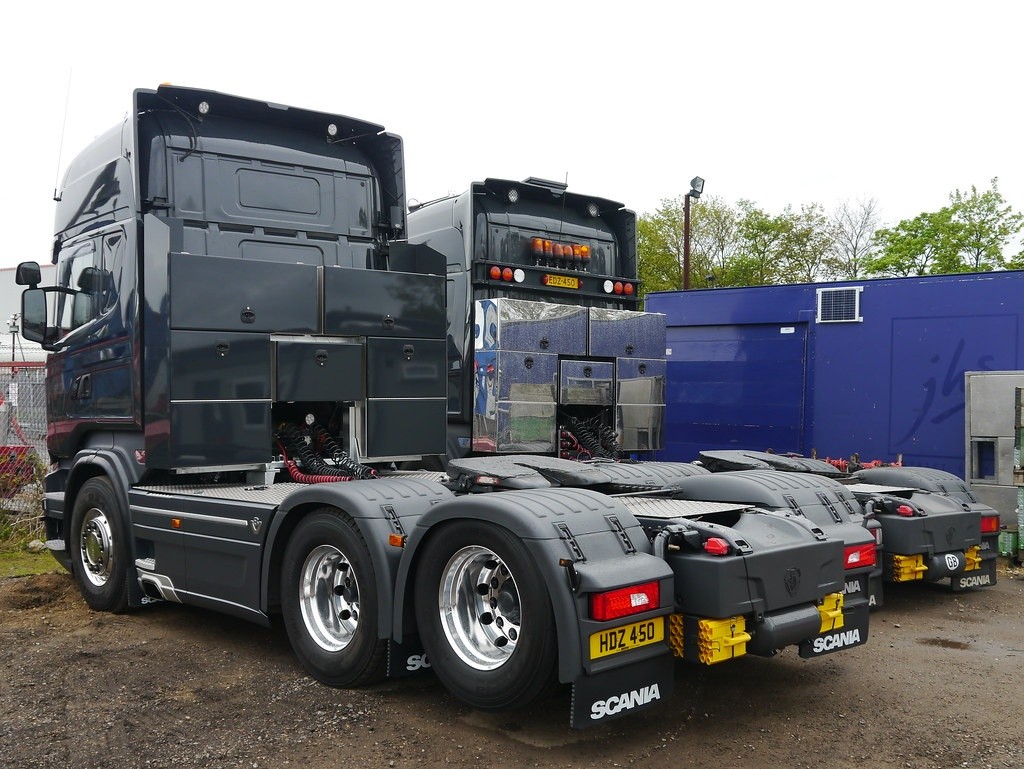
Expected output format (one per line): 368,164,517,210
14,85,879,735
407,176,999,609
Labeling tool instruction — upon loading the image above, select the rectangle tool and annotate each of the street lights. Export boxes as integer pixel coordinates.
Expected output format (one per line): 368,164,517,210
682,177,704,291
11,326,19,375
